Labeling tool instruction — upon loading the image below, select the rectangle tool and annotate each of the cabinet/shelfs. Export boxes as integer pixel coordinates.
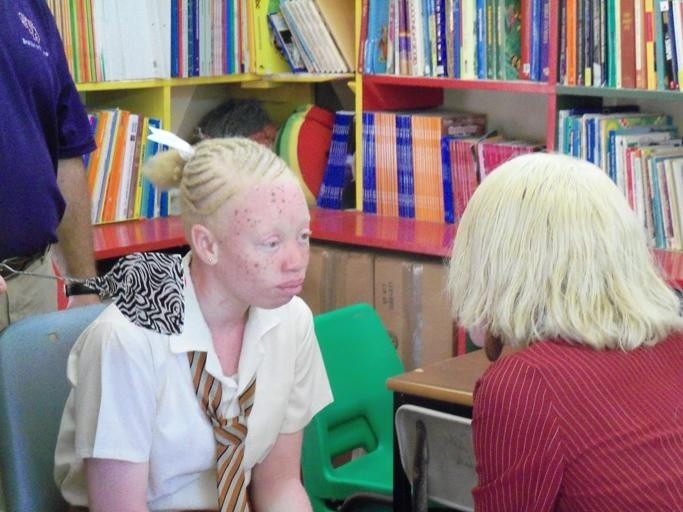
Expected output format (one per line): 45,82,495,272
41,1,683,292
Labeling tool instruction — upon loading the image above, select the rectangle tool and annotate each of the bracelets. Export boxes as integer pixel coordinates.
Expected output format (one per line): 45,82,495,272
63,277,100,297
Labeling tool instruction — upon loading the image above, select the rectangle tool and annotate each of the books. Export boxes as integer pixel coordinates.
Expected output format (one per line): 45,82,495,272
78,99,190,227
364,2,679,90
169,0,361,81
558,108,680,248
317,111,356,210
361,110,548,225
37,1,170,88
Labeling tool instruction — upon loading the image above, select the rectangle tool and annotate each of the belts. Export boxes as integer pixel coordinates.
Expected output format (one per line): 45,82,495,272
0,254,42,279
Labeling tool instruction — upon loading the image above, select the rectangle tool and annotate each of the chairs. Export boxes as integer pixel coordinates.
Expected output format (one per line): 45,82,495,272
300,300,406,511
1,302,111,511
395,403,478,511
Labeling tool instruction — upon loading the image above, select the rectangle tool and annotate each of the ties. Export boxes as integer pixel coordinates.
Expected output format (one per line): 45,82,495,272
189,354,255,512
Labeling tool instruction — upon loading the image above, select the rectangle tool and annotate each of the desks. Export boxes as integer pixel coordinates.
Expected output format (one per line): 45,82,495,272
385,345,497,510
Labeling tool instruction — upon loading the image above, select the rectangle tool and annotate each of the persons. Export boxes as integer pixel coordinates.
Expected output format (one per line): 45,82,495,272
53,126,334,512
444,150,683,512
0,0,108,329
